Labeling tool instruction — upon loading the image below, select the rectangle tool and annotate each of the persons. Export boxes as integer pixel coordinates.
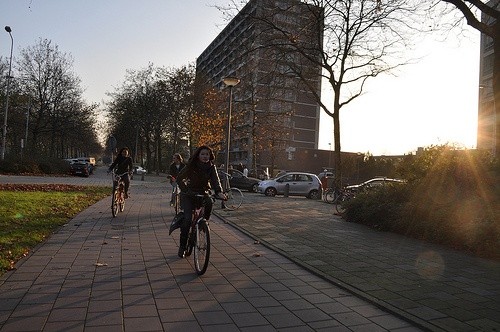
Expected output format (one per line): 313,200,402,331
107,146,133,209
212,162,227,208
167,153,187,207
322,174,327,195
216,160,282,181
176,145,228,259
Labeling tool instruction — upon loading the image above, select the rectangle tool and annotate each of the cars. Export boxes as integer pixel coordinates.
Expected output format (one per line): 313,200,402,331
316,171,335,188
135,165,147,176
257,171,323,201
63,157,95,177
217,169,262,194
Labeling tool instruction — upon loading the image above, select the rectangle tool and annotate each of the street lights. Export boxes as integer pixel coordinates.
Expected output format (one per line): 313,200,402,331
221,76,241,198
328,142,331,150
0,24,14,161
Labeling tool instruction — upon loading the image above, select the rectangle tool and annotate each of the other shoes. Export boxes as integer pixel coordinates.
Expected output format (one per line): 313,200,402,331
178,244,187,259
124,193,129,199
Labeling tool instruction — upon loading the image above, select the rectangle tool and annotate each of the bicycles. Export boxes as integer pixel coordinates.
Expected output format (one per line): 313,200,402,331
185,191,230,275
205,171,244,211
107,170,134,218
169,179,188,217
324,183,374,217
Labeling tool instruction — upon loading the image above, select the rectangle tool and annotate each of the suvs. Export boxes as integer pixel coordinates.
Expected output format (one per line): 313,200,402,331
344,177,408,194
